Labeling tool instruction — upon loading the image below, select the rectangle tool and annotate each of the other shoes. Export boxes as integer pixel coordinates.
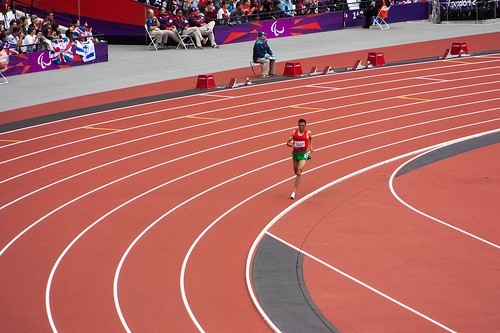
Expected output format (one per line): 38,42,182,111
212,44,219,48
291,192,295,199
363,25,369,28
198,46,204,49
358,15,365,19
202,37,208,44
270,74,277,76
263,74,266,77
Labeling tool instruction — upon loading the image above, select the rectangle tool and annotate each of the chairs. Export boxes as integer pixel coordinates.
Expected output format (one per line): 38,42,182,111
175,29,196,50
370,5,391,30
0,66,9,85
144,24,159,51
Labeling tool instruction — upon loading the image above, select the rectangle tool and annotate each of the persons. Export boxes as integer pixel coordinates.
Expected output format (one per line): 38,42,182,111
287,119,313,200
188,7,221,48
156,6,187,49
137,0,330,25
173,9,208,50
355,0,391,28
146,9,169,49
0,0,94,55
253,31,276,78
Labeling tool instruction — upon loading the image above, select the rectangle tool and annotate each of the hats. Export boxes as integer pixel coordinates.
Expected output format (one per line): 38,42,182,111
191,7,197,12
148,10,154,13
258,32,265,36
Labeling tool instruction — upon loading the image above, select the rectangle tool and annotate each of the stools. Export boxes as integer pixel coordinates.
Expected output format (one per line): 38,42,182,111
249,61,263,76
